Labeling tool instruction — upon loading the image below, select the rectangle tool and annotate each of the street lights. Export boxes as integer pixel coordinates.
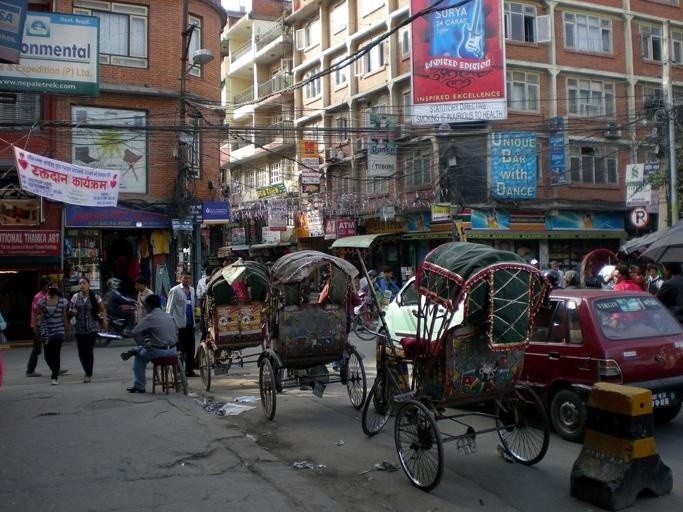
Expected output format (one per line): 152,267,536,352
174,49,214,286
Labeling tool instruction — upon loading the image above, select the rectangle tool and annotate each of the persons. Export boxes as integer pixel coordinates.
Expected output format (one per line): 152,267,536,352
103,277,137,336
120,294,178,394
67,277,107,383
366,266,400,331
196,266,217,340
359,269,376,294
134,275,155,322
25,277,68,378
530,259,662,298
515,245,533,263
165,271,200,377
655,262,682,326
32,280,68,385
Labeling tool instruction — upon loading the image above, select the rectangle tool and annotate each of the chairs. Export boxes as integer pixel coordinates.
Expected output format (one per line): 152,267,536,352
393,287,465,400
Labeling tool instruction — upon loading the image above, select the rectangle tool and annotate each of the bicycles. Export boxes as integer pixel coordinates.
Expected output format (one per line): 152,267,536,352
349,291,408,341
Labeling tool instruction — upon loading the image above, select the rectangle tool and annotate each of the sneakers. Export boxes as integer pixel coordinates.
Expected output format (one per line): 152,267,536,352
51,379,58,384
58,370,67,374
26,373,41,377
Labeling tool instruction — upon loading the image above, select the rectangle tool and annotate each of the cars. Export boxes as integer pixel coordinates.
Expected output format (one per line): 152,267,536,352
514,290,682,440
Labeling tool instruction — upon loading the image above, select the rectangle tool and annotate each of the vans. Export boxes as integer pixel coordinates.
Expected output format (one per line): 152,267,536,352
374,276,467,353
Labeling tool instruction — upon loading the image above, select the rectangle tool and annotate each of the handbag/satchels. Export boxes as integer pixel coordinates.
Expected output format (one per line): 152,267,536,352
70,293,79,326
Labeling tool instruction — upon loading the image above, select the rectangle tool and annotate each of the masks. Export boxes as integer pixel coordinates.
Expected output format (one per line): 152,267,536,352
48,288,58,295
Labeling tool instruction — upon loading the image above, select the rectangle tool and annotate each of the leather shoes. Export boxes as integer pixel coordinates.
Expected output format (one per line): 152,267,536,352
121,349,137,361
84,376,91,383
126,386,145,393
186,372,200,377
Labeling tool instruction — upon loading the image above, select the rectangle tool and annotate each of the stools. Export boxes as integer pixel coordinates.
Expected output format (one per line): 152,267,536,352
149,356,178,394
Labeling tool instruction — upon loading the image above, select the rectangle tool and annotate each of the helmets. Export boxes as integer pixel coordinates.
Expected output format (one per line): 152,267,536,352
544,270,559,285
107,277,122,289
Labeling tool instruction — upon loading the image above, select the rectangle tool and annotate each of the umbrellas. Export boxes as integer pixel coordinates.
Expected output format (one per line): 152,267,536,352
637,229,682,264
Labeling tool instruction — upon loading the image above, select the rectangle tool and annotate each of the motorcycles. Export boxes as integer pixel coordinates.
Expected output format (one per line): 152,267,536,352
95,304,138,346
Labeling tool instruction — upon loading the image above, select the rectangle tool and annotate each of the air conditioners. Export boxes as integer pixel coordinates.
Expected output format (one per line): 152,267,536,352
393,123,410,142
358,136,365,153
325,148,335,160
604,121,621,139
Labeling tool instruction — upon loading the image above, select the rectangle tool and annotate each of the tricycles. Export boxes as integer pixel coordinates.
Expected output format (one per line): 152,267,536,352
256,250,367,421
357,242,550,490
197,257,270,392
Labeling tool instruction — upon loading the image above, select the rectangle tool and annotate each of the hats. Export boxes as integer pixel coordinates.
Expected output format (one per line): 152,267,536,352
562,270,579,282
530,259,538,264
368,270,377,276
384,266,392,271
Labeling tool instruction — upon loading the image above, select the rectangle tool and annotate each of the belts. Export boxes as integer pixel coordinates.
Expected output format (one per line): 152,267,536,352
152,346,174,349
